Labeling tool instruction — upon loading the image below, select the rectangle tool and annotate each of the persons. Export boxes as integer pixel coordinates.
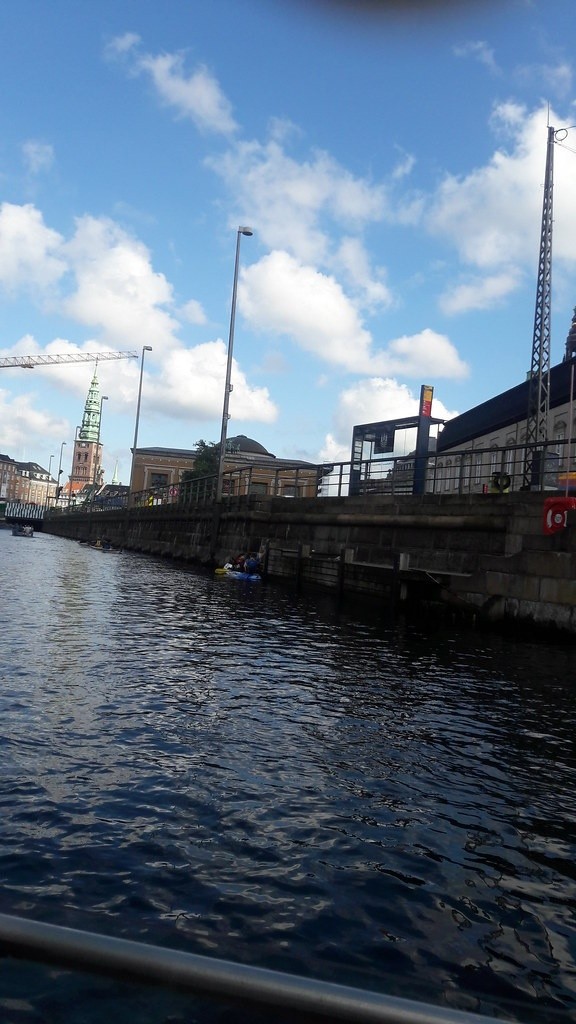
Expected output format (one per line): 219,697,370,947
71,491,77,505
234,552,262,573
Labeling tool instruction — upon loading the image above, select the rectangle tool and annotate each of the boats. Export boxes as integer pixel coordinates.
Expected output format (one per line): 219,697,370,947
78,540,123,555
212,566,263,585
11,525,34,537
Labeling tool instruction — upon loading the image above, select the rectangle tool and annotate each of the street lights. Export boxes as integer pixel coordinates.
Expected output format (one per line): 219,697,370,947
90,395,109,512
125,345,153,510
68,426,81,509
55,442,66,508
44,455,55,507
215,225,254,502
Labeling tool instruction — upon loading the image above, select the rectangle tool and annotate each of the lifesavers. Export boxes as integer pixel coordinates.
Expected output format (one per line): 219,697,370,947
170,487,179,496
495,474,511,489
